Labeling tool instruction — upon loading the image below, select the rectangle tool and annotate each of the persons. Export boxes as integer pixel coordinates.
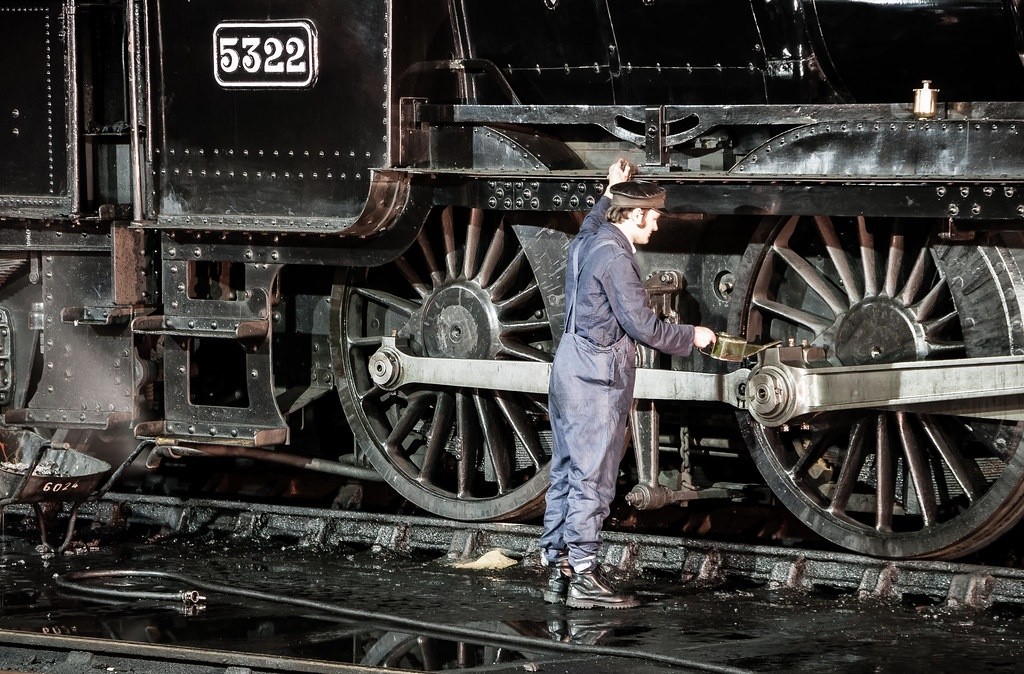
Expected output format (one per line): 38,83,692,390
539,157,717,609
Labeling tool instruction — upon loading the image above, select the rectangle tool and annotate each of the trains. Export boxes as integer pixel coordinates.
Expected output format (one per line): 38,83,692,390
0,0,1024,566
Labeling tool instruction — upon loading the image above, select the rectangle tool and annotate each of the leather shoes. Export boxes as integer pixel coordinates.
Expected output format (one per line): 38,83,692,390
544,566,571,604
566,573,641,609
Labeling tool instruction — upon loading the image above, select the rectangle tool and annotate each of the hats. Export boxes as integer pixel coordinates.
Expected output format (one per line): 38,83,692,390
610,180,666,209
